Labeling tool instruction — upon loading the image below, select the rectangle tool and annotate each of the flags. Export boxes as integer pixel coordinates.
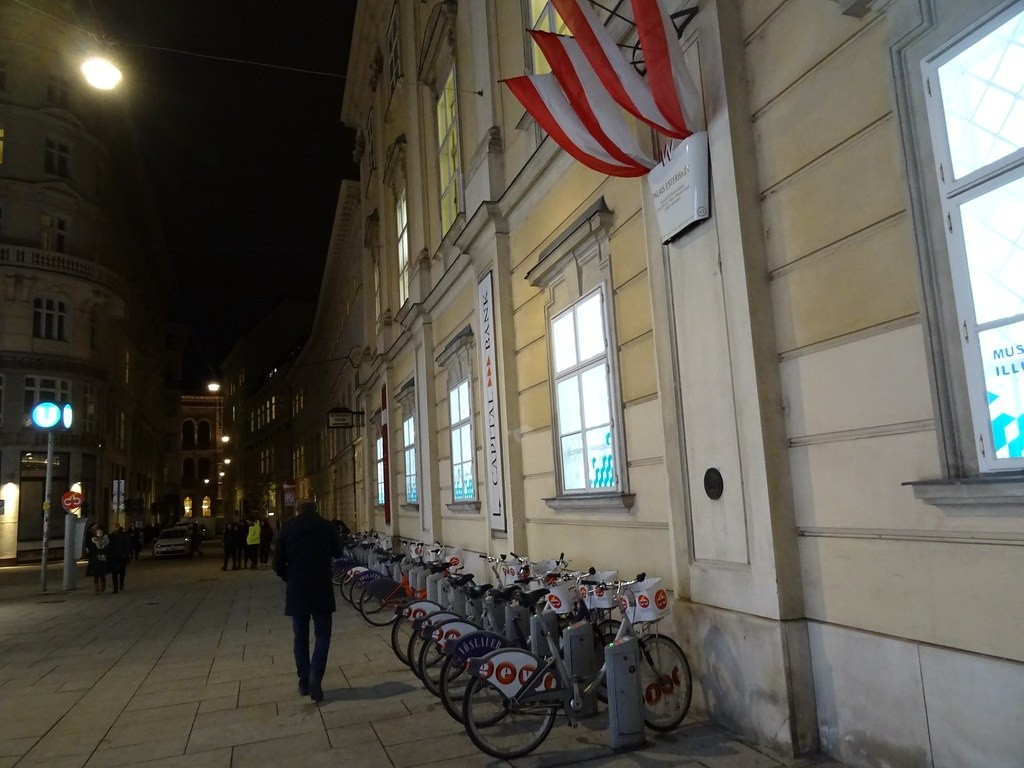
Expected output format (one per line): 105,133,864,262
503,0,705,180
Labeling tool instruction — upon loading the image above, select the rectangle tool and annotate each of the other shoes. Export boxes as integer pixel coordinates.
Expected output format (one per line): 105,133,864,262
119,581,124,590
297,677,310,697
111,590,119,594
307,674,324,702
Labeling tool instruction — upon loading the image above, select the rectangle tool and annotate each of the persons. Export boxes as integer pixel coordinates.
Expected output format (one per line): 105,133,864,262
190,514,275,573
86,520,167,595
272,497,345,701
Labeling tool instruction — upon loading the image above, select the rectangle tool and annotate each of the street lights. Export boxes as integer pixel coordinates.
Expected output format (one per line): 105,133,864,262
29,399,74,594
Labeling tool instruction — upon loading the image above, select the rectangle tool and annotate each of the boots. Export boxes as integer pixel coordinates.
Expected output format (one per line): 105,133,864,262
101,584,105,593
94,584,99,595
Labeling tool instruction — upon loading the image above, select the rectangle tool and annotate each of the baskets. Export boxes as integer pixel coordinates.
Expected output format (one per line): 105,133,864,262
443,546,465,572
544,571,580,614
495,557,529,588
529,561,562,585
420,546,445,562
577,570,618,609
617,576,671,624
406,542,424,558
379,536,393,551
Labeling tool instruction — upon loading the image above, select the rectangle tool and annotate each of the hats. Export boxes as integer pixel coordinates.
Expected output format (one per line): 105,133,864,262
111,522,120,531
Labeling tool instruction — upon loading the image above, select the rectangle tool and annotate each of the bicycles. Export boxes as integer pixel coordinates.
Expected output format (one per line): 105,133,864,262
329,525,693,761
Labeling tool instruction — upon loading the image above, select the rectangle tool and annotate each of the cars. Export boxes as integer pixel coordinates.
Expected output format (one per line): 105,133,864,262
173,521,206,543
151,527,192,560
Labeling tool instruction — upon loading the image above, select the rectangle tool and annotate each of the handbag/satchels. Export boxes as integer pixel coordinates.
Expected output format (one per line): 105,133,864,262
98,551,106,561
246,520,261,545
